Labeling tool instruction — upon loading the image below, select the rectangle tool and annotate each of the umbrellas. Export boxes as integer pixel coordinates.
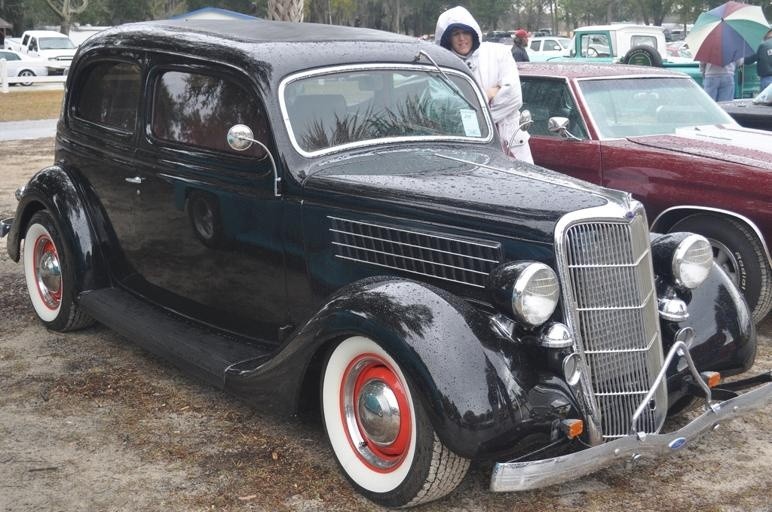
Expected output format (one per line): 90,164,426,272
684,0,771,67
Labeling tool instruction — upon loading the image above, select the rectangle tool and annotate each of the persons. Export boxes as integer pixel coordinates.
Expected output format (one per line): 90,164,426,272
758,28,772,92
699,57,744,101
511,29,530,62
428,6,534,164
